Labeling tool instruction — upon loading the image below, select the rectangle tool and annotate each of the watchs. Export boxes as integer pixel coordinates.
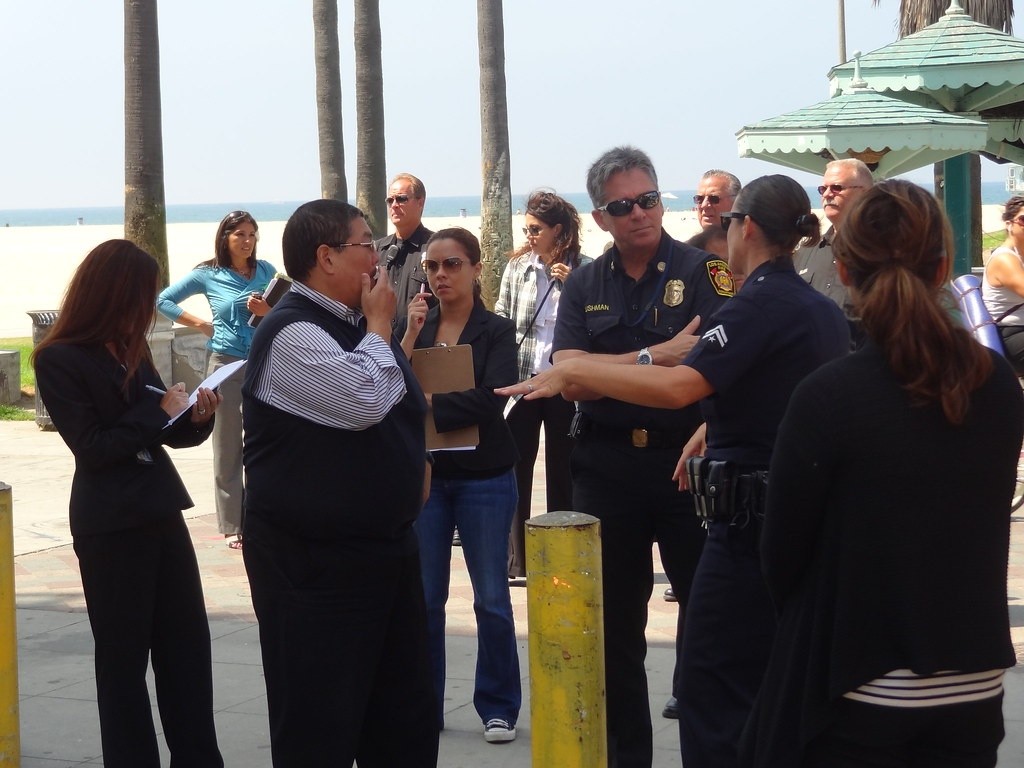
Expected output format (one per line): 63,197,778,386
636,346,653,365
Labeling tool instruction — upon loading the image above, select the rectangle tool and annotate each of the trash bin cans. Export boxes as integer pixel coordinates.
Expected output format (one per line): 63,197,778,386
26,309,62,432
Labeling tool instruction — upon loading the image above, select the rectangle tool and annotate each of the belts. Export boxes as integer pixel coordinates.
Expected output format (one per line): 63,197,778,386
586,422,695,450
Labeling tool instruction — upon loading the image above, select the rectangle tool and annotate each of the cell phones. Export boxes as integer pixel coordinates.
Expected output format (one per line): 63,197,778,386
252,295,262,301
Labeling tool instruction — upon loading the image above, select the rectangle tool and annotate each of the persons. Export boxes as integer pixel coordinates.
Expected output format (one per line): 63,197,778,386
154,210,278,549
373,172,437,335
550,144,737,768
738,175,1024,768
494,185,596,579
665,170,741,603
27,238,226,768
241,198,435,768
492,175,854,767
662,228,747,720
400,226,522,742
792,157,873,340
978,195,1024,378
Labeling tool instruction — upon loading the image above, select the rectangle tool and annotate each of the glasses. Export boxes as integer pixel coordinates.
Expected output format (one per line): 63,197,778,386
522,223,557,236
693,195,737,204
223,211,253,238
420,258,478,275
818,184,867,195
385,195,422,205
1008,215,1024,226
597,190,661,216
313,240,377,264
720,212,763,231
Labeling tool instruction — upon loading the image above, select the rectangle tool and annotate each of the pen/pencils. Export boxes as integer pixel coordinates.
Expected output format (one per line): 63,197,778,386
146,385,166,395
418,283,426,324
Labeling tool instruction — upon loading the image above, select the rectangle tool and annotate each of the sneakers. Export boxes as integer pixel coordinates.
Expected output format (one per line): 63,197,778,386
483,718,516,743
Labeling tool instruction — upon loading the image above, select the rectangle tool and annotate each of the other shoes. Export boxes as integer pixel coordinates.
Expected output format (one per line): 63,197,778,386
663,588,679,602
662,696,680,719
452,525,462,547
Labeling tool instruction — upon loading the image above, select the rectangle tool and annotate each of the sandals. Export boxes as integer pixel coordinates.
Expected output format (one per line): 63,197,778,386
224,534,242,549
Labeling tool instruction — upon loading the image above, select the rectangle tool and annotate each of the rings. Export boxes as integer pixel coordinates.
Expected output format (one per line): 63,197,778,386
527,385,533,392
199,409,206,415
177,383,181,391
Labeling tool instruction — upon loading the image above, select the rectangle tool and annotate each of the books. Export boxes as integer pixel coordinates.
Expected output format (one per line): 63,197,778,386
246,272,293,329
